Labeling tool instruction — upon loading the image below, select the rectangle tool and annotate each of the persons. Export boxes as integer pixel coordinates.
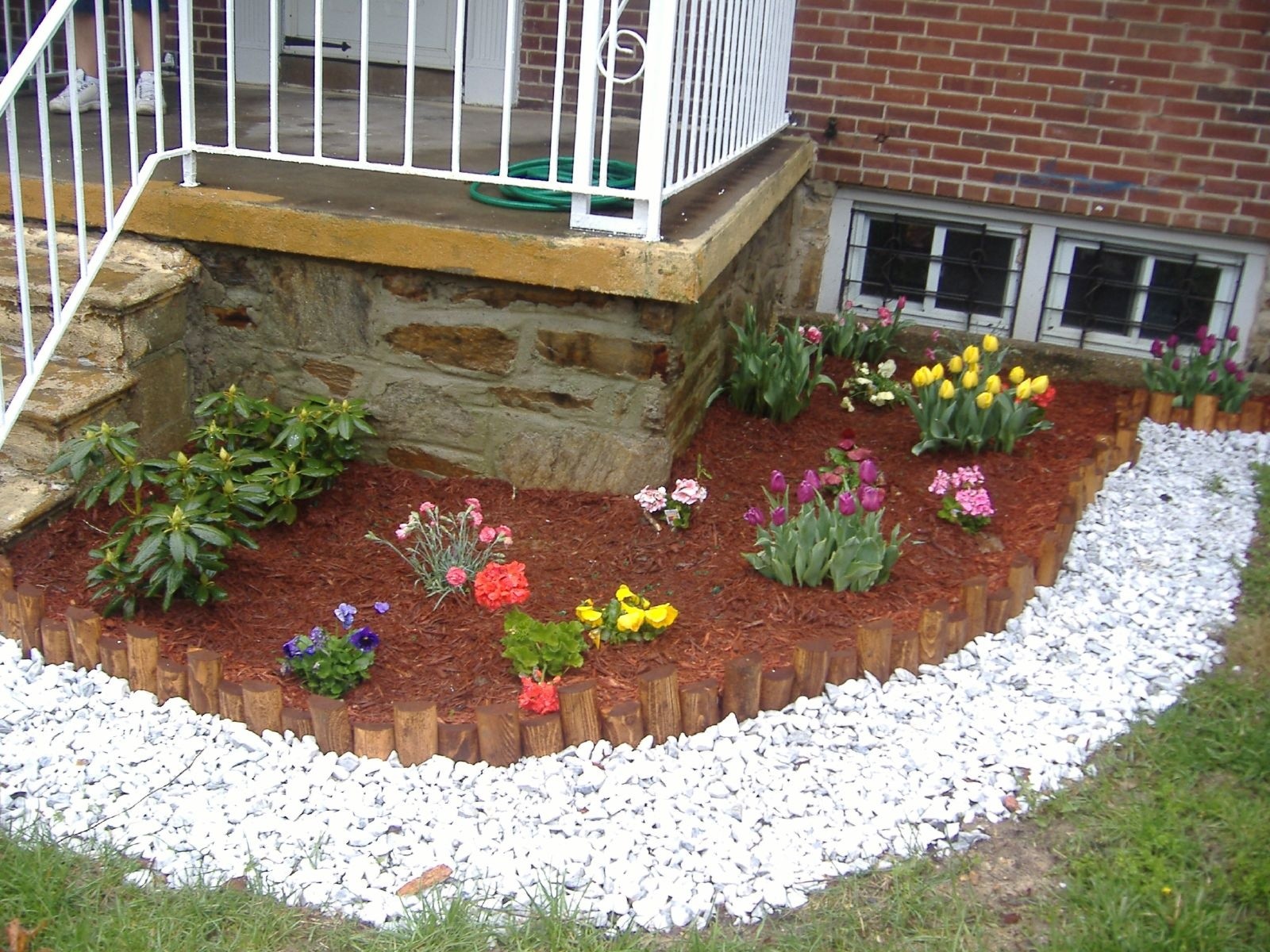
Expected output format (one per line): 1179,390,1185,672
44,0,168,117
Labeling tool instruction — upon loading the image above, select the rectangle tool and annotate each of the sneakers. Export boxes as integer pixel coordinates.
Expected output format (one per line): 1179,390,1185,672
135,71,168,116
48,68,112,113
